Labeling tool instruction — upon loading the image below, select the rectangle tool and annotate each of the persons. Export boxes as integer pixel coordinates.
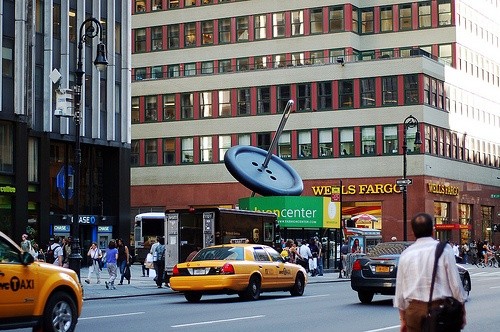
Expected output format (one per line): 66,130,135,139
104,239,119,289
142,238,149,277
48,236,73,267
21,234,44,261
118,239,130,285
187,247,201,261
85,243,102,284
393,213,467,332
150,238,169,288
341,240,348,272
352,239,361,253
281,237,323,276
450,238,500,268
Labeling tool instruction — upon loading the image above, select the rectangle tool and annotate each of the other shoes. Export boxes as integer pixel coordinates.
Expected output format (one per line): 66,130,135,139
96,281,101,284
84,279,91,284
111,286,117,289
128,280,131,284
104,280,110,290
118,281,123,285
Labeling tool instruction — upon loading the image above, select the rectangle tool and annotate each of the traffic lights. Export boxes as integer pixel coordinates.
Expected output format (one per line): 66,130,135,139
492,223,499,232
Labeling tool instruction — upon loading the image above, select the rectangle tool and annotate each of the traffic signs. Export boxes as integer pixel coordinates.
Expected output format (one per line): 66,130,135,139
396,178,413,185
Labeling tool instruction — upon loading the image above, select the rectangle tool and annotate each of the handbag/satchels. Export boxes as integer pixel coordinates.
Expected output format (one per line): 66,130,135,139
125,265,131,279
308,258,319,270
146,253,155,269
420,298,466,332
87,257,93,266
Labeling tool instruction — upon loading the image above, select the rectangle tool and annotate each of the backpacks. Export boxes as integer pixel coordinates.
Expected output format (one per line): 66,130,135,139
45,245,60,264
281,248,291,261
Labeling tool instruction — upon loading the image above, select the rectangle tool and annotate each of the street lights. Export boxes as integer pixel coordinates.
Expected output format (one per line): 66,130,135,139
67,18,110,283
402,114,423,241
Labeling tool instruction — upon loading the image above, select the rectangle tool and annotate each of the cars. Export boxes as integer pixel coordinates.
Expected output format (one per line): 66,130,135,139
0,231,84,332
169,243,309,304
351,240,471,304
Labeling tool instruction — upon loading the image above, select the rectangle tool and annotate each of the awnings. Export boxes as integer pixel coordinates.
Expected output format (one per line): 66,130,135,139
434,224,472,229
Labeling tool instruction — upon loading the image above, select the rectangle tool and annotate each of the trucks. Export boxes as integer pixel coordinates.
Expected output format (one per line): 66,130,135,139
163,207,280,277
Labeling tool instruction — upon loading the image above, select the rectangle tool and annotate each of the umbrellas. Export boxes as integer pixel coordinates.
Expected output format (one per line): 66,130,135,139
351,214,378,228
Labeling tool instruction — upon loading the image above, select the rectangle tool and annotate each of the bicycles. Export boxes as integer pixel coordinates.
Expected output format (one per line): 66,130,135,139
476,251,500,269
